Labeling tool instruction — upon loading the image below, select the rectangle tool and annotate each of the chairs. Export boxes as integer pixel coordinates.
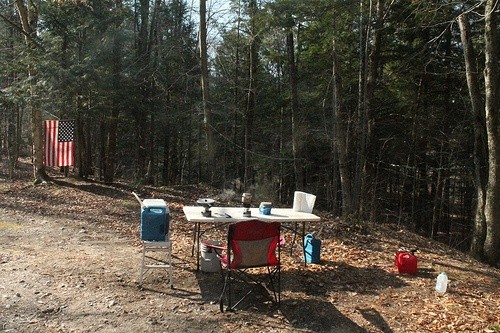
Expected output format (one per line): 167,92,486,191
203,220,285,314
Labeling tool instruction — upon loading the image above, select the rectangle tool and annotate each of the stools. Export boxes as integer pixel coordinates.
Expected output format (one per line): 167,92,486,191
137,238,174,289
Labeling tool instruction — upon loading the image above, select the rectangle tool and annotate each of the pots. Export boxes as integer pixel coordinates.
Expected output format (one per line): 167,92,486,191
197,197,215,208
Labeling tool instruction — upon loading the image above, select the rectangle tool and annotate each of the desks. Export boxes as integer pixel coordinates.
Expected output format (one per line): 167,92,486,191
182,204,322,275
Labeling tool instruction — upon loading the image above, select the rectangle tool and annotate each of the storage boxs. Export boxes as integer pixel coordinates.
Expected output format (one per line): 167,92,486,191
140,199,171,242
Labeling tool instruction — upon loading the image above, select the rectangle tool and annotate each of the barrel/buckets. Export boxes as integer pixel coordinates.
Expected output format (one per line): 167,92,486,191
304,233,321,264
398,252,418,274
201,240,222,273
141,206,167,242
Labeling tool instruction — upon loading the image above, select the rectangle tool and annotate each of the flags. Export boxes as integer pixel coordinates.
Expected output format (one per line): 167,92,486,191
41,119,75,166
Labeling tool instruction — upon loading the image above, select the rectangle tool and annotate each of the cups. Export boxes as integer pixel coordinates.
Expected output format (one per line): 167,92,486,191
259,205,272,215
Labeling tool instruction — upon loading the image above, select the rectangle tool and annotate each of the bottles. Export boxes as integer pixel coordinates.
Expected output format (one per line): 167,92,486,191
436,272,448,292
